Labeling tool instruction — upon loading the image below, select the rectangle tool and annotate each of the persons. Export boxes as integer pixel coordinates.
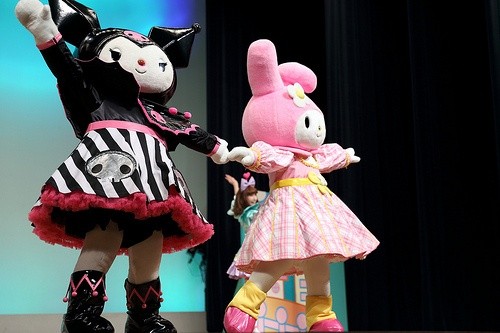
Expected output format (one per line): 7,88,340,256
222,171,264,298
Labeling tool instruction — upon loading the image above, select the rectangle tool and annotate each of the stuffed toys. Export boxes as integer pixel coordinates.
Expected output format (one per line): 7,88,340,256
222,37,381,333
15,0,231,333
